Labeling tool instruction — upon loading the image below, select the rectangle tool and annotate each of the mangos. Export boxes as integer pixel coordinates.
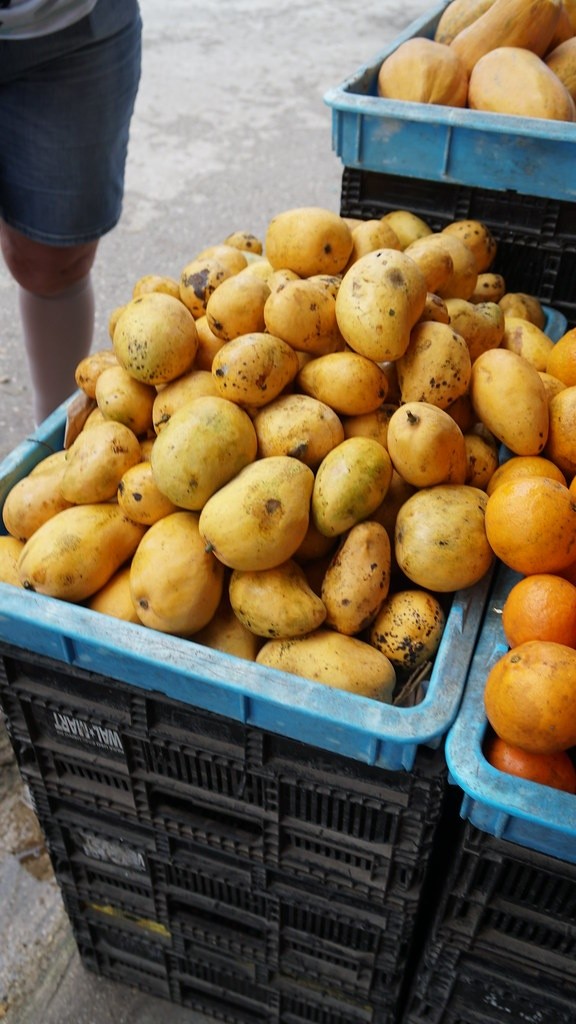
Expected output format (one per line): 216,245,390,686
0,205,549,714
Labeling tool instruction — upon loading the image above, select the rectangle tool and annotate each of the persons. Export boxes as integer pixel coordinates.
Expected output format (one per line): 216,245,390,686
0,0,144,427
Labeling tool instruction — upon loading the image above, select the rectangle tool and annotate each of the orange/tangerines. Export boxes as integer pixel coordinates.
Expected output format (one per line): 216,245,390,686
484,319,576,795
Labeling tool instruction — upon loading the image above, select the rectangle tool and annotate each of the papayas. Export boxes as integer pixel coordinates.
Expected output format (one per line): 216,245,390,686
375,0,576,125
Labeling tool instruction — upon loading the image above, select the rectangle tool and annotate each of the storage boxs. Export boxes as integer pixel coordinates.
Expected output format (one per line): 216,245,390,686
323,0,576,330
405,554,575,1024
0,381,490,1024
